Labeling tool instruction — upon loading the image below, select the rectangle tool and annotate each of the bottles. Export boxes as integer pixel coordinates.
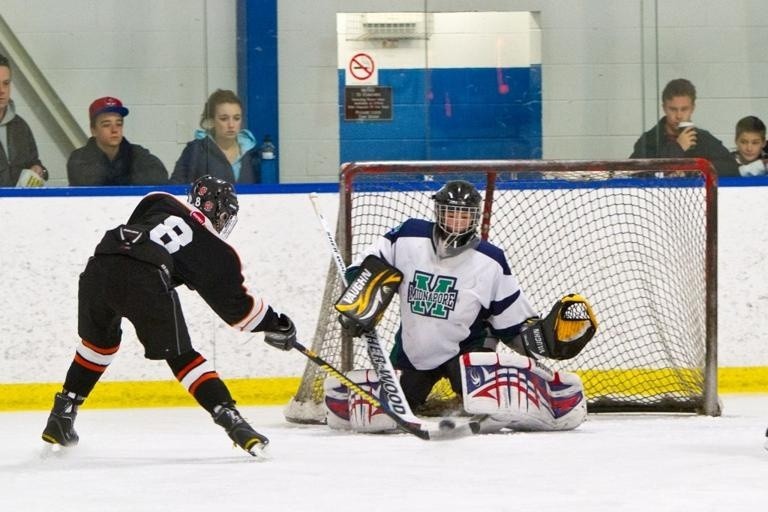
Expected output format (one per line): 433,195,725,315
260,134,276,184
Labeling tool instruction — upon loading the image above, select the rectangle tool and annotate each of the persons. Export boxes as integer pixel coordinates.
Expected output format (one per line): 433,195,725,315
65,95,169,185
731,114,768,176
36,173,296,458
321,176,588,432
628,79,741,178
169,88,262,185
0,54,49,187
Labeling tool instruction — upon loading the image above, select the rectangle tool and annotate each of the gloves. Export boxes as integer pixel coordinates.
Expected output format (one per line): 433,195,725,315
263,312,297,352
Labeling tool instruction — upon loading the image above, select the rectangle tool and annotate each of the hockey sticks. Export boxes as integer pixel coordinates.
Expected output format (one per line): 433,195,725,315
309,192,454,432
293,341,479,440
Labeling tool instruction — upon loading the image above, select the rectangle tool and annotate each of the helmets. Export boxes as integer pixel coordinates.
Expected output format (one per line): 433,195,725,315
431,181,482,249
186,174,239,239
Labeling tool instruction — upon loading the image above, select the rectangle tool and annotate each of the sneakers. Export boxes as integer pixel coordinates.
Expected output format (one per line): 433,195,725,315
213,404,270,454
41,392,80,448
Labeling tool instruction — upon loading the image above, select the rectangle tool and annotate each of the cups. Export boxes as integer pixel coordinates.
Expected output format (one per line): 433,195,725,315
678,121,696,151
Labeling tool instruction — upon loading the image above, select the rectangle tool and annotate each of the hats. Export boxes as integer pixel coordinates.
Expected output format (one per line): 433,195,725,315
89,96,129,121
